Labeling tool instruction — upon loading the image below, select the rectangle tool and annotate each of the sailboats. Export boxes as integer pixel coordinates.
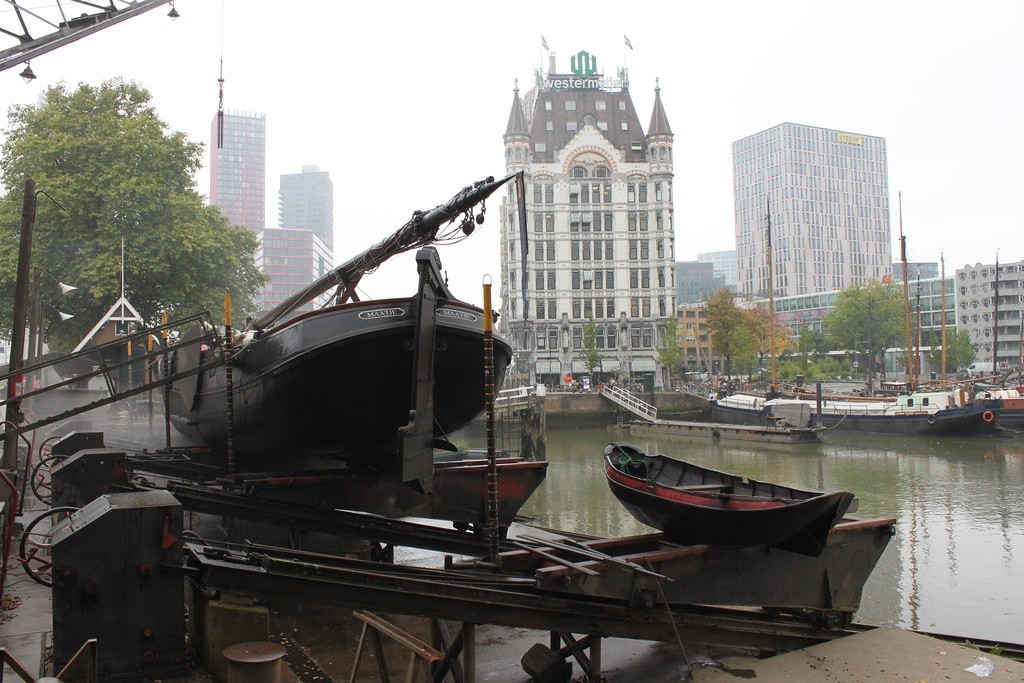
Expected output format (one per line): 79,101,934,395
690,245,1024,425
708,187,1004,437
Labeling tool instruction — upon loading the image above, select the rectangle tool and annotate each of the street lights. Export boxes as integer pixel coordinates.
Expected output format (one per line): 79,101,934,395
33,297,75,396
26,264,80,411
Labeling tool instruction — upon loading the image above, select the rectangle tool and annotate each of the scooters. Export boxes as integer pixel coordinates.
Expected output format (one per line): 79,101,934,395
569,381,584,393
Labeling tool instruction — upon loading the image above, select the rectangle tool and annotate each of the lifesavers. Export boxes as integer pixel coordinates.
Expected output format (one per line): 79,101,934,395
927,415,936,425
982,411,994,423
713,430,720,438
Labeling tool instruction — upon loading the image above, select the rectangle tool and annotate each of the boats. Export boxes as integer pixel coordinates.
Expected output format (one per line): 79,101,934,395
603,442,860,559
146,168,530,453
